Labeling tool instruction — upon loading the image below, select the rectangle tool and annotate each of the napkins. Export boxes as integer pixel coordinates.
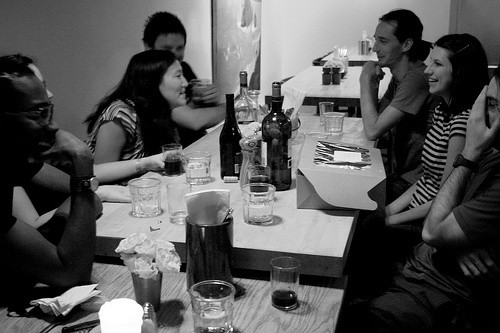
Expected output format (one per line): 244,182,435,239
31,283,103,315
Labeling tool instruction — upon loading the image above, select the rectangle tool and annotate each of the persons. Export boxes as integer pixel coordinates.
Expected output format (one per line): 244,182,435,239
141,11,241,148
358,8,440,179
0,53,153,298
82,50,189,181
369,66,500,333
383,34,489,259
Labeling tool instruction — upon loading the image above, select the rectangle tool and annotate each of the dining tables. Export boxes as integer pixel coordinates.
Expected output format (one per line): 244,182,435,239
313,42,391,66
265,65,393,118
0,260,345,333
84,114,384,279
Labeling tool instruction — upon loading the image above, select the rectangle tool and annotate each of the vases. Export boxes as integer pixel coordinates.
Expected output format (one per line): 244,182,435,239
130,270,162,308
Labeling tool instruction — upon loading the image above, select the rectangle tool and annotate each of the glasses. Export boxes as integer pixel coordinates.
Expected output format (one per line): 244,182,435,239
0,104,53,126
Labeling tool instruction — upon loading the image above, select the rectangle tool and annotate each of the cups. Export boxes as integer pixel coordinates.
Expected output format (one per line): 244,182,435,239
182,151,211,185
241,183,276,226
162,144,183,176
319,101,333,125
269,256,300,311
165,182,192,225
339,56,348,74
359,40,369,56
324,112,345,135
185,215,234,292
128,178,162,218
247,165,271,198
190,280,236,333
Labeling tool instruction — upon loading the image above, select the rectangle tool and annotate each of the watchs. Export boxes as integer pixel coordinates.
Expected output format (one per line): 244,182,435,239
452,153,476,171
69,174,99,194
135,157,148,176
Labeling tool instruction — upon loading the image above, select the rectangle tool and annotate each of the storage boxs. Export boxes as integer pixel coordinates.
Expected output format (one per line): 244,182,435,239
298,133,388,213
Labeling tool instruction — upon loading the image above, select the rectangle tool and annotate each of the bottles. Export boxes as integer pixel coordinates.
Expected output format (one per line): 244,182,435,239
141,303,157,333
233,70,257,137
322,68,331,84
332,67,341,85
219,93,243,181
261,81,291,191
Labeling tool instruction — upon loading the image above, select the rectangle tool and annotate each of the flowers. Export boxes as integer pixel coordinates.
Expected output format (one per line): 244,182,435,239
114,233,181,280
